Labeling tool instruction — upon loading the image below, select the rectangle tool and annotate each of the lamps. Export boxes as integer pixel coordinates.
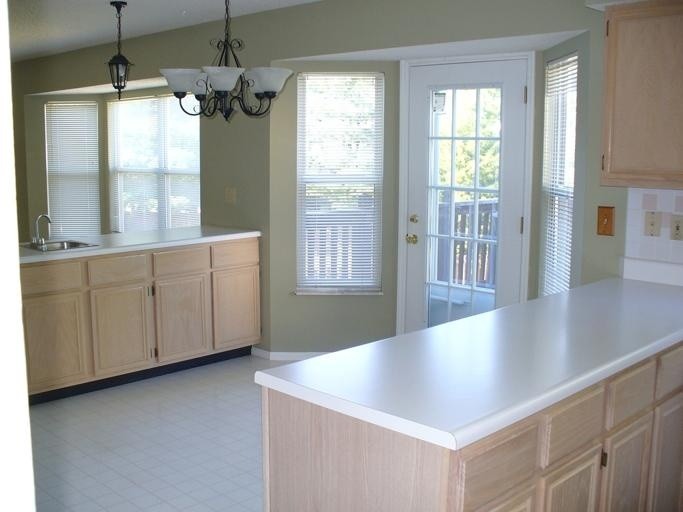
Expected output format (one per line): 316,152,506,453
104,0,135,101
159,0,294,121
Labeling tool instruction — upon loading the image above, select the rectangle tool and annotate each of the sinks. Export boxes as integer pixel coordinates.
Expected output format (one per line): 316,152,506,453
19,240,101,255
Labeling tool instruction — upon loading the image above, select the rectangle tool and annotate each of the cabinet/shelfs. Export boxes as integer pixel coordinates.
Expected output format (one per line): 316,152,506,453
599,0,683,190
261,340,683,512
18,237,261,396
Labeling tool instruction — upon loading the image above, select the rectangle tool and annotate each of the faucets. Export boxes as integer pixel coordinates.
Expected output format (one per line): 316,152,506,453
34,213,53,246
